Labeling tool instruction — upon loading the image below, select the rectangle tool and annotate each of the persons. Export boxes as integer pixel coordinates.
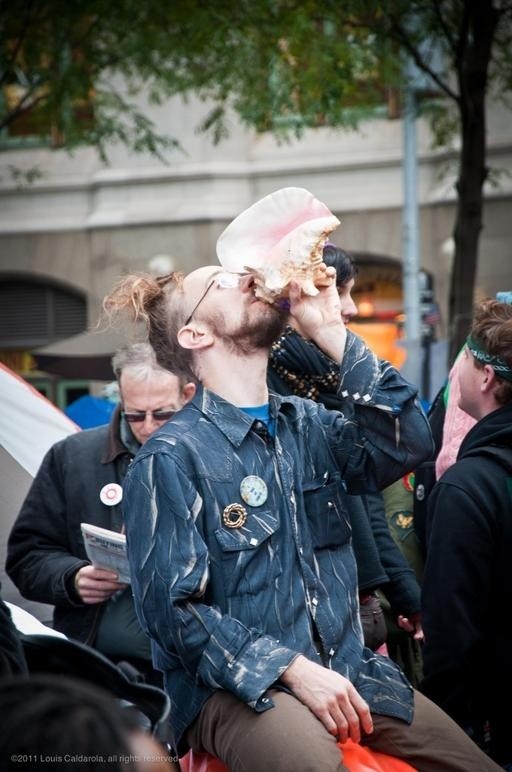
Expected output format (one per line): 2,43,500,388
5,343,207,771
262,246,426,657
1,671,180,771
414,295,511,770
105,245,511,770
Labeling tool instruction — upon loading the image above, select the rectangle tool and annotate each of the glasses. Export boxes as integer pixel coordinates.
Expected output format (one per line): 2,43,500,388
120,406,177,422
184,272,242,327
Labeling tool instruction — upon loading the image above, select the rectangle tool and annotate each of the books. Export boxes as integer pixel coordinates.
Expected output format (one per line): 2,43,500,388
81,521,133,588
